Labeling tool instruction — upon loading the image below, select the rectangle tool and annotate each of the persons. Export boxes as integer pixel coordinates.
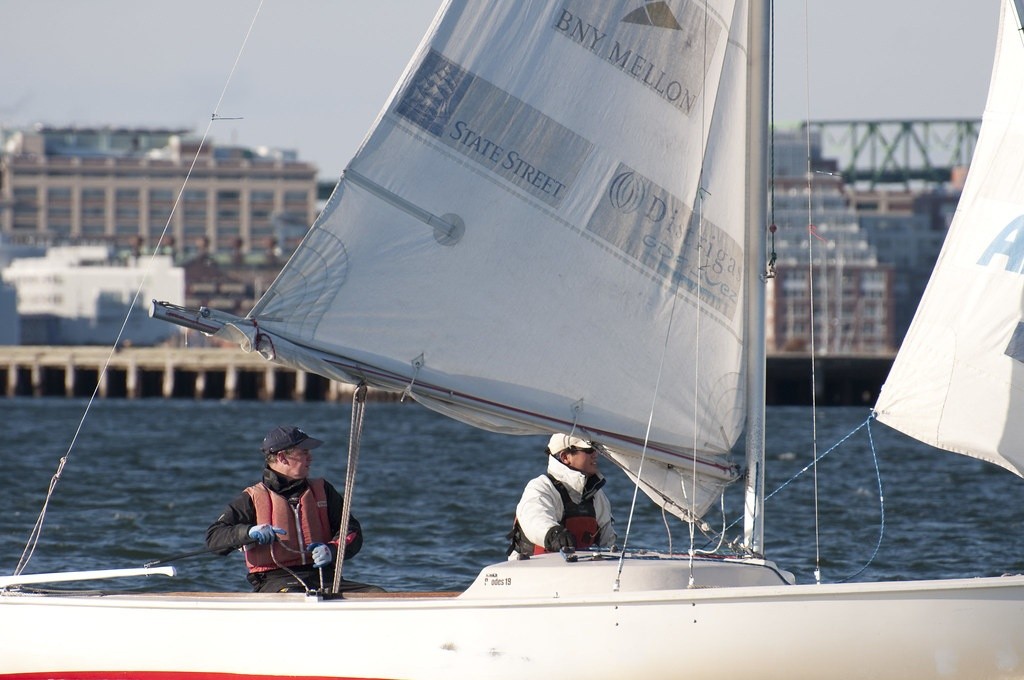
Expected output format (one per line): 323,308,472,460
505,433,619,562
206,426,388,595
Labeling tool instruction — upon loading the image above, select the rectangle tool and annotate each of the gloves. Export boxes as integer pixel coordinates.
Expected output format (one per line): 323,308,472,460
249,524,287,546
306,542,333,567
546,526,576,553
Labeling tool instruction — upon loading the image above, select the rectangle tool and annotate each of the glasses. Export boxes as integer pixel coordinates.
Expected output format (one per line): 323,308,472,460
572,446,599,454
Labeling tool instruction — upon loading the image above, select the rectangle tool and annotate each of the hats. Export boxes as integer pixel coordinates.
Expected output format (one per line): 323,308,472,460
548,432,592,454
262,427,323,453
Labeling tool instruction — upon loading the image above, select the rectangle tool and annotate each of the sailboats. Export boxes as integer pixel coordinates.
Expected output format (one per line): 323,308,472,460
0,0,1024,680
